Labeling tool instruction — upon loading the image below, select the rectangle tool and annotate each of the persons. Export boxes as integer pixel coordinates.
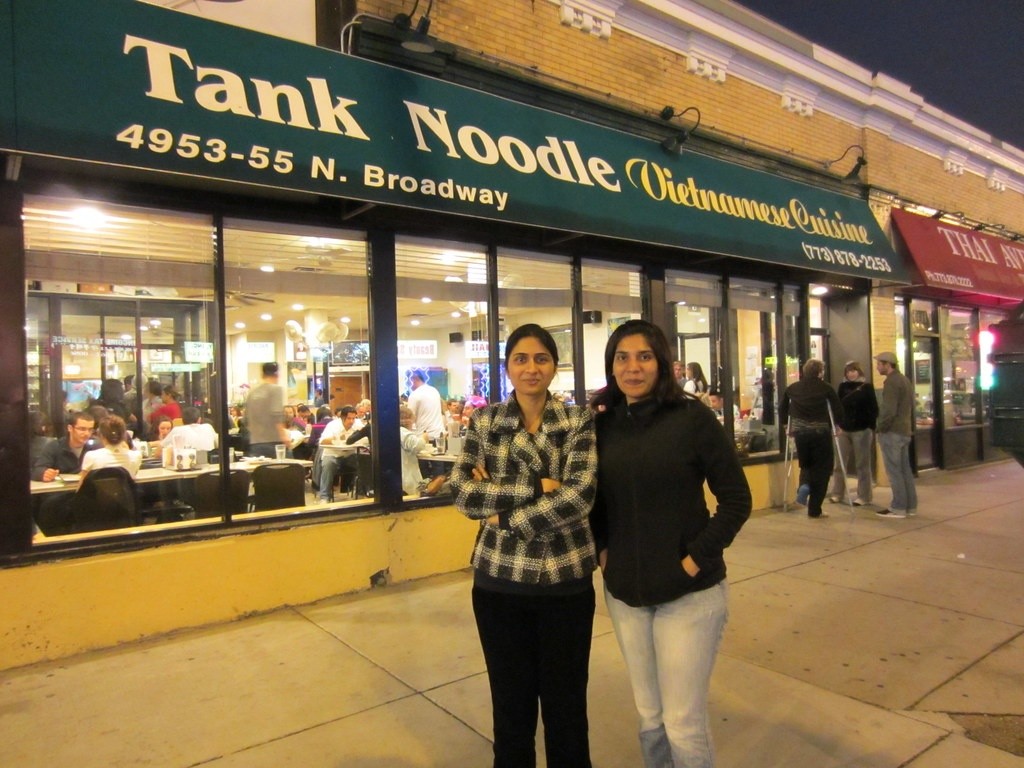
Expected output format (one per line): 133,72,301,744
778,358,844,520
828,360,880,507
29,412,95,482
407,368,448,480
28,362,489,537
449,323,598,768
74,414,142,494
673,361,741,423
589,319,753,768
241,362,294,459
873,352,918,518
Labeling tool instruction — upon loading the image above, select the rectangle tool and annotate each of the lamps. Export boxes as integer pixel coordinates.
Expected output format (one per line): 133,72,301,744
824,145,867,185
395,0,435,53
661,106,701,154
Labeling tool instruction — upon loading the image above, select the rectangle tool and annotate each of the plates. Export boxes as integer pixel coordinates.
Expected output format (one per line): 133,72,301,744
416,453,432,456
57,473,81,482
240,458,271,464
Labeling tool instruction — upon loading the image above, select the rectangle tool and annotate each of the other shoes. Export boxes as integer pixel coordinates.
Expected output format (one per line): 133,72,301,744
796,484,810,505
829,496,842,502
906,508,918,516
808,511,829,518
876,509,907,518
320,497,330,504
851,499,867,506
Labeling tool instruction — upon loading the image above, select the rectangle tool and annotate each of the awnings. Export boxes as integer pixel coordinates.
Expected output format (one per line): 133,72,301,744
0,0,912,286
890,208,1024,302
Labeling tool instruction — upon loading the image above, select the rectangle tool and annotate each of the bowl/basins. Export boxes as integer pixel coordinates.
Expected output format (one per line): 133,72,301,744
422,444,433,450
331,440,346,445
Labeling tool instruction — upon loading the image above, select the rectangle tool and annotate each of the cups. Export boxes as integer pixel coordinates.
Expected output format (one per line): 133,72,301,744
305,423,312,436
435,437,445,453
275,444,286,460
229,448,234,462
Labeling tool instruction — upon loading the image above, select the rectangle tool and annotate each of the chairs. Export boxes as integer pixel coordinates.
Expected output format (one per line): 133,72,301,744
252,463,306,512
72,467,143,531
195,469,249,518
356,446,374,500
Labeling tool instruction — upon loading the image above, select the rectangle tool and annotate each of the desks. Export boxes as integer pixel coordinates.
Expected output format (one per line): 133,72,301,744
417,451,457,479
319,443,367,500
30,467,183,514
167,459,313,479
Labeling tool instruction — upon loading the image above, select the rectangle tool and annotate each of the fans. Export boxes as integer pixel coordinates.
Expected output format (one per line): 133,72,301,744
283,318,350,344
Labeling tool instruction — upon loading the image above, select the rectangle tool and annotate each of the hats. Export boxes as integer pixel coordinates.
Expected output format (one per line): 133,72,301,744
874,352,898,365
